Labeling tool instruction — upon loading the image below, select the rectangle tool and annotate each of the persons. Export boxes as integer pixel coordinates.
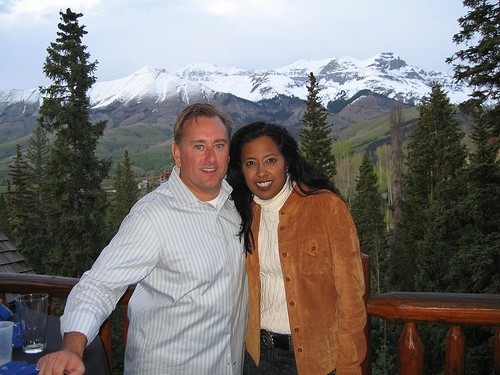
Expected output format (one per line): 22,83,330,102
225,121,367,375
38,103,249,375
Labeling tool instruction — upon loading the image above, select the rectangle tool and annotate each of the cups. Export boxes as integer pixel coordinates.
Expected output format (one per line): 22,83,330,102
15,293,49,354
0,321,14,367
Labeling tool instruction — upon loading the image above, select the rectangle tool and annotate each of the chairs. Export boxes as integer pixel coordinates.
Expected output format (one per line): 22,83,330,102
46,314,111,375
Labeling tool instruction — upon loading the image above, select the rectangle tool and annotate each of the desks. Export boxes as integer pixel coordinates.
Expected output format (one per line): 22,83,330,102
12,346,47,364
